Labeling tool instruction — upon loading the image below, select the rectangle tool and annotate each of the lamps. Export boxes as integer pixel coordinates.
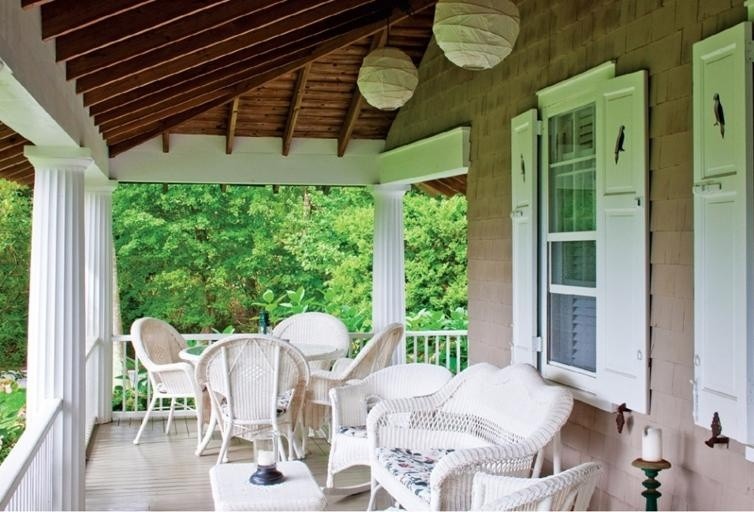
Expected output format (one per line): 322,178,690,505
358,47,419,110
432,0,519,71
318,362,454,505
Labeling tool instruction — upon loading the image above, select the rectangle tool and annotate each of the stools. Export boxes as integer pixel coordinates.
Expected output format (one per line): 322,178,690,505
209,459,327,511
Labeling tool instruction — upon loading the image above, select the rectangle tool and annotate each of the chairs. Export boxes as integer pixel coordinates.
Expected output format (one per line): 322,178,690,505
364,362,574,511
272,312,349,438
301,322,405,457
470,461,607,511
194,334,311,464
130,317,204,448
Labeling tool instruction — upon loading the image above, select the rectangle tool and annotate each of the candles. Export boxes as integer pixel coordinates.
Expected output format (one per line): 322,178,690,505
248,449,285,488
632,426,673,461
